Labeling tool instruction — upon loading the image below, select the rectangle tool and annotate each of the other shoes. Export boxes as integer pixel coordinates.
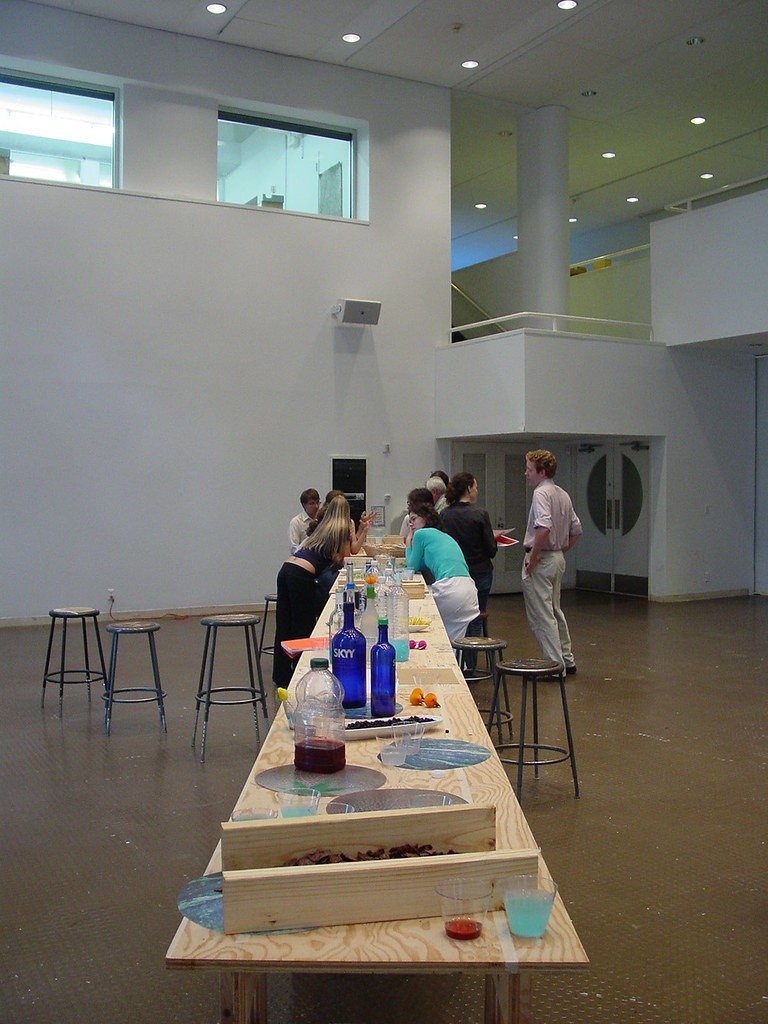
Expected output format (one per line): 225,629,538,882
566,665,577,674
527,675,566,683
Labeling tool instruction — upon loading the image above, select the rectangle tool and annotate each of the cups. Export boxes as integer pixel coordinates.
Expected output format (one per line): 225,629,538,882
282,698,296,730
391,722,426,755
307,802,355,818
406,794,453,809
498,874,558,936
376,729,410,766
434,876,493,939
230,807,279,822
275,787,321,818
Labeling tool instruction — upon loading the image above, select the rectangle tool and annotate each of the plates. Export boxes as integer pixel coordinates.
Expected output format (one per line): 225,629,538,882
408,624,428,633
343,714,443,740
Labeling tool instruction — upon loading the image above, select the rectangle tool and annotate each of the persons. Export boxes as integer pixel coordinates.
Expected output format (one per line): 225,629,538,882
520,450,583,680
404,502,482,671
272,494,352,695
439,472,498,670
289,488,377,587
397,471,454,543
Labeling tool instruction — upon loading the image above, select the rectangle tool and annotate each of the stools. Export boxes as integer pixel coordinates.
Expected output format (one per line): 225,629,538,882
258,594,278,667
450,609,583,806
104,621,169,737
194,613,271,764
42,605,108,701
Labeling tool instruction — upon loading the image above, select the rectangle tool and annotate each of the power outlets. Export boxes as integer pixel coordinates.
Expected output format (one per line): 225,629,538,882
107,589,115,602
704,573,711,582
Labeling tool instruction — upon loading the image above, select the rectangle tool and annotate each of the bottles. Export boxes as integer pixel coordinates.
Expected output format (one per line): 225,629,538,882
293,658,345,773
331,601,367,709
327,559,409,662
370,617,396,717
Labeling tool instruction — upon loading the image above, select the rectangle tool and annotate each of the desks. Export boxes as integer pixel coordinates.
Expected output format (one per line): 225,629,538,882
164,551,592,1024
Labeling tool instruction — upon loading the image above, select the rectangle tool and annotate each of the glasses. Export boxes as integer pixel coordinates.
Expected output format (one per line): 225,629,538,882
305,501,321,506
